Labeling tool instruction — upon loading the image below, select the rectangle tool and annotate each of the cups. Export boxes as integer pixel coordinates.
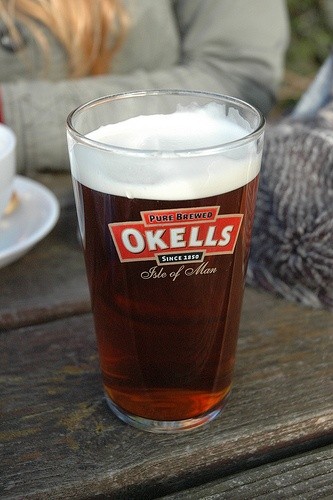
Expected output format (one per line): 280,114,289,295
66,88,268,433
1,124,18,214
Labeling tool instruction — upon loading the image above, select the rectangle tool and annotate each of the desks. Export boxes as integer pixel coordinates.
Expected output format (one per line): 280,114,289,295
1,168,330,500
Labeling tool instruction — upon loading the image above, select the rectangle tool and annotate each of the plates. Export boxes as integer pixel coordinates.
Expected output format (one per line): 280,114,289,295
0,174,61,268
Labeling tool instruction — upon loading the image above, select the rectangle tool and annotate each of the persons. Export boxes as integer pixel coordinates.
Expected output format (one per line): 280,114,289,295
0,0,291,175
